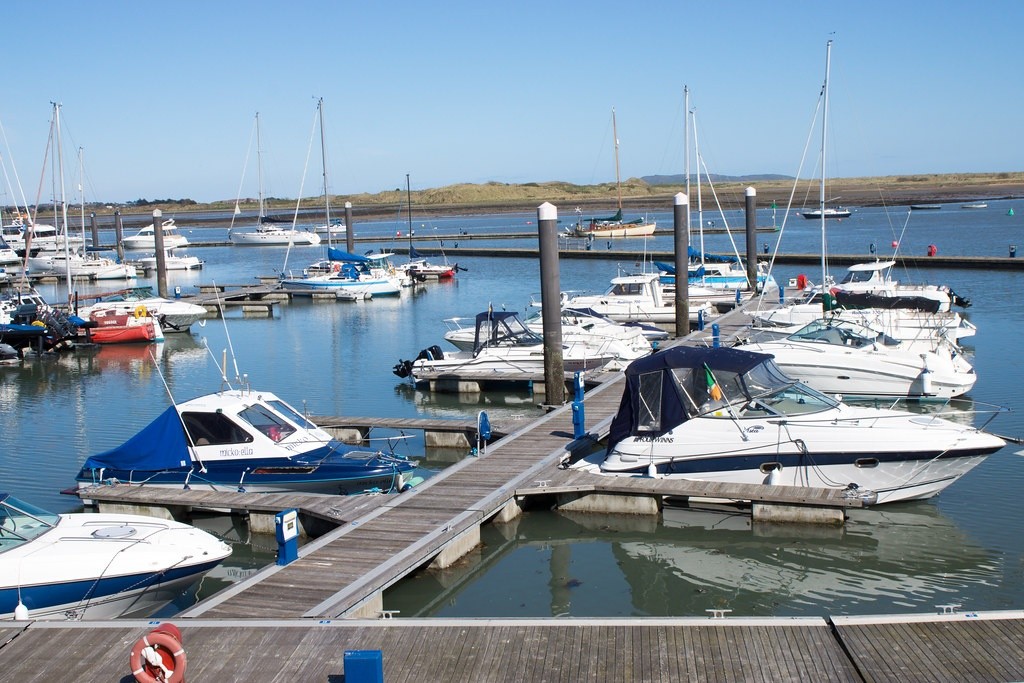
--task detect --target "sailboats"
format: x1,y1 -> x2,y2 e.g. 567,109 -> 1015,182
687,40 -> 976,339
390,172 -> 468,279
615,83 -> 778,290
226,111 -> 323,246
0,101 -> 163,367
802,148 -> 850,219
278,96 -> 402,296
565,105 -> 658,237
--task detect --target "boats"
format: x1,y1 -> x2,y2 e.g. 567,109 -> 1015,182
530,275 -> 714,323
961,204 -> 987,209
565,345 -> 1012,507
139,244 -> 202,271
393,303 -> 653,381
911,205 -> 942,210
356,252 -> 425,286
100,286 -> 207,332
122,217 -> 190,249
803,260 -> 973,312
75,373 -> 418,507
0,492 -> 234,622
314,219 -> 347,233
733,317 -> 977,404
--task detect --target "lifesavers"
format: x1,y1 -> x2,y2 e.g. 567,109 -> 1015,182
129,633 -> 185,683
31,321 -> 46,328
870,243 -> 876,253
607,241 -> 612,249
134,306 -> 146,320
